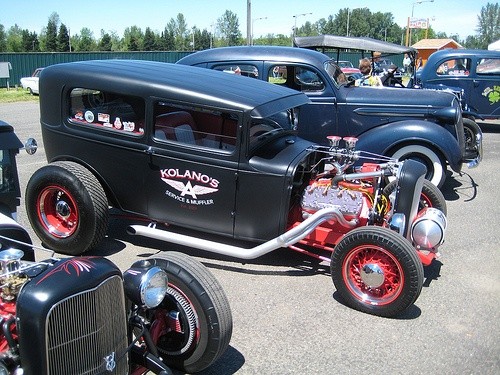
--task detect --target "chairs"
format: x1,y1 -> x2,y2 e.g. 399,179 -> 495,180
136,111 -> 204,146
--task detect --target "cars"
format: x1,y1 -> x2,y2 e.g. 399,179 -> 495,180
222,63 -> 291,81
175,45 -> 469,191
414,44 -> 500,123
20,67 -> 84,96
330,60 -> 360,72
0,118 -> 236,374
25,59 -> 450,318
477,59 -> 499,72
380,59 -> 405,72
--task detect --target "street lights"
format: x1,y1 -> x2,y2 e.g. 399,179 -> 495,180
292,15 -> 296,48
408,0 -> 435,47
346,7 -> 351,36
294,13 -> 313,38
67,25 -> 73,52
384,27 -> 388,42
251,16 -> 267,46
191,25 -> 197,51
210,24 -> 213,49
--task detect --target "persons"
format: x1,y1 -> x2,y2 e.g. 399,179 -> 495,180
273,66 -> 280,79
417,57 -> 423,71
372,52 -> 384,72
234,65 -> 241,75
354,57 -> 382,86
402,54 -> 411,68
